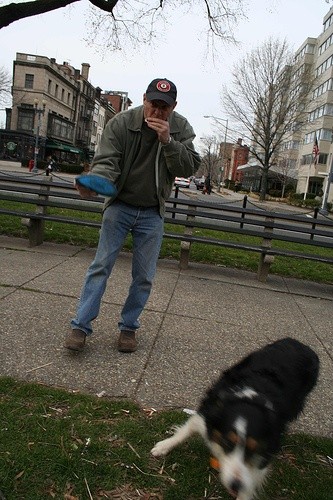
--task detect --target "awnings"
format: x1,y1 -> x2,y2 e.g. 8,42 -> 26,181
48,140 -> 80,153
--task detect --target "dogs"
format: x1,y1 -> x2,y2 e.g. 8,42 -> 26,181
150,337 -> 320,500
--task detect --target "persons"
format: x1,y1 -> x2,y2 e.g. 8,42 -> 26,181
204,177 -> 210,193
48,157 -> 54,173
63,78 -> 202,352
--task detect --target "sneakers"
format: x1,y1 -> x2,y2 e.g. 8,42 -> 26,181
118,330 -> 137,352
66,329 -> 86,352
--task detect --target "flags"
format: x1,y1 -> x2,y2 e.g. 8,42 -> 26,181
313,139 -> 320,155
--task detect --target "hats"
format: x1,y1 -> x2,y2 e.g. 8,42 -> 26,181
146,78 -> 177,106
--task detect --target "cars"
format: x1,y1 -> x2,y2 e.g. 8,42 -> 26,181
174,176 -> 191,188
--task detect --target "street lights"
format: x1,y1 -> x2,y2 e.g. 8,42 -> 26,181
30,96 -> 47,173
202,115 -> 229,194
